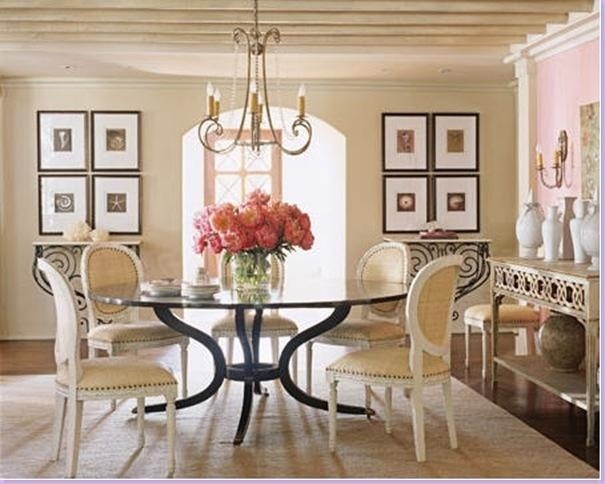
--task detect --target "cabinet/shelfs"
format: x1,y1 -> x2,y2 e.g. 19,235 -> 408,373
485,256 -> 600,447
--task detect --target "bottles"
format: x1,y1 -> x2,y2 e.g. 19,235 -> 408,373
514,196 -> 599,273
192,267 -> 211,286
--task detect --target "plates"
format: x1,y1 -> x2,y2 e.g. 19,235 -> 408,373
137,279 -> 221,300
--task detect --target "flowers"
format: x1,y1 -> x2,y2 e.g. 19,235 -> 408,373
190,188 -> 314,287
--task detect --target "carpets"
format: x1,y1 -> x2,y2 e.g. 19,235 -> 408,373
1,374 -> 600,478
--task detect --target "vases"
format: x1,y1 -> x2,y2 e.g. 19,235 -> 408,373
516,201 -> 546,258
569,200 -> 591,263
557,197 -> 579,259
581,201 -> 600,271
541,205 -> 564,261
230,253 -> 272,299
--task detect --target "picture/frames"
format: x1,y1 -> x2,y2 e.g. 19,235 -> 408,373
382,112 -> 429,172
432,174 -> 480,233
432,112 -> 479,172
90,110 -> 142,172
92,174 -> 142,235
382,174 -> 430,234
36,110 -> 89,172
38,174 -> 90,236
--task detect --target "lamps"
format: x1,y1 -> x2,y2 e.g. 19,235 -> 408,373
197,0 -> 313,155
535,129 -> 568,189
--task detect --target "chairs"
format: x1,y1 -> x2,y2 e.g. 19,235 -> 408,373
212,253 -> 299,388
325,253 -> 458,463
79,244 -> 189,408
304,242 -> 411,405
33,257 -> 178,479
